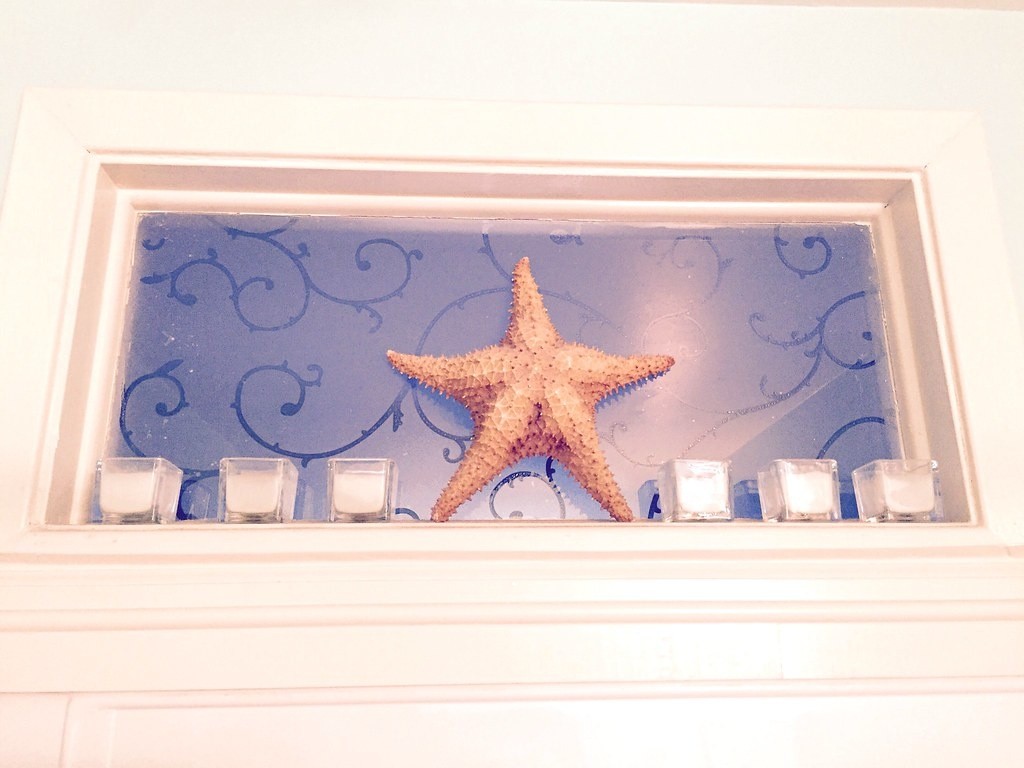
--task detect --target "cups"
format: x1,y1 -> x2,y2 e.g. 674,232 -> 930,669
657,458 -> 735,522
851,458 -> 942,523
327,458 -> 398,524
99,457 -> 183,524
217,458 -> 299,524
756,459 -> 842,521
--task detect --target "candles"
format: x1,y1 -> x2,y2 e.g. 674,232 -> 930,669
99,458 -> 182,523
851,459 -> 942,523
216,457 -> 299,523
326,459 -> 398,523
656,459 -> 731,523
757,459 -> 843,522
